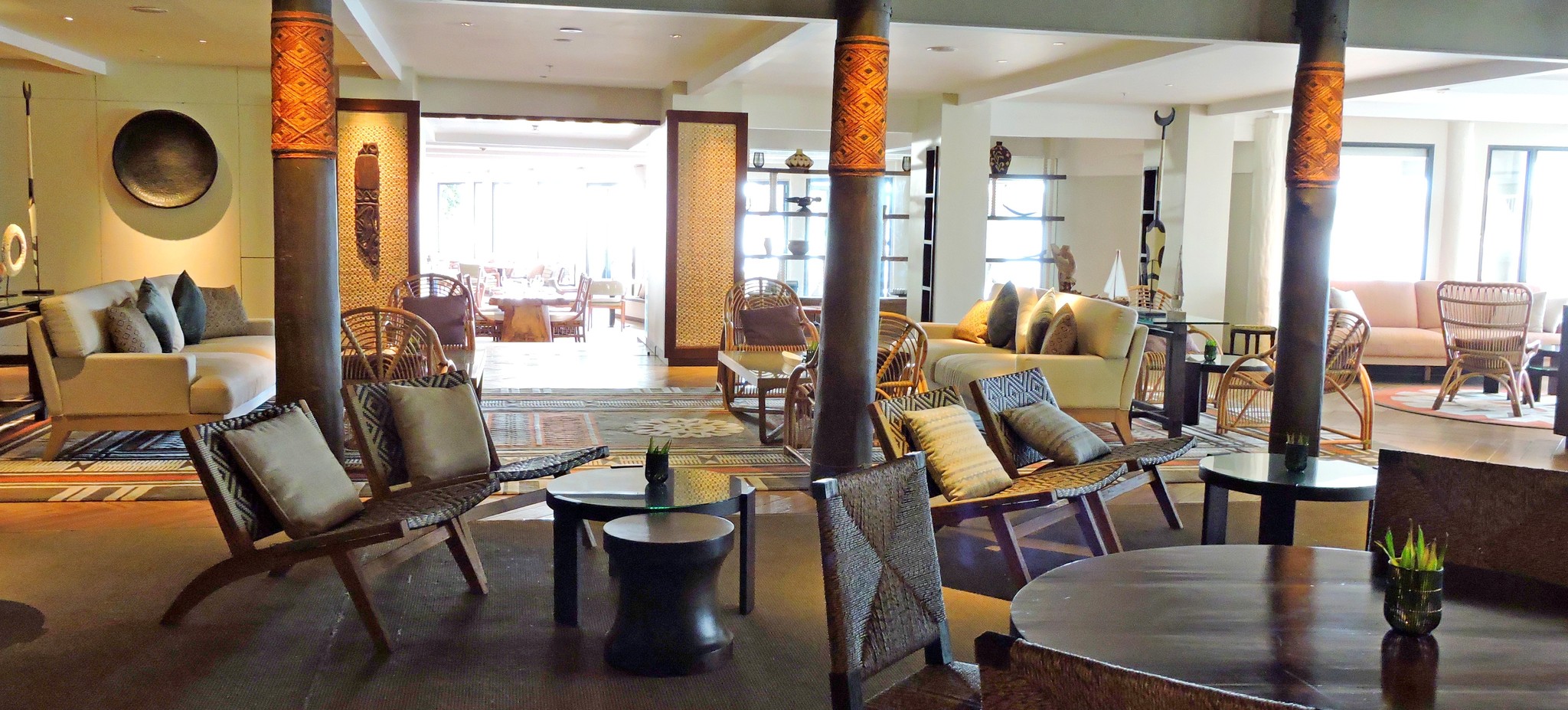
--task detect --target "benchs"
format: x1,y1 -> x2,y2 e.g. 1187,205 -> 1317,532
27,270 -> 276,461
1326,281 -> 1568,383
891,280 -> 1149,445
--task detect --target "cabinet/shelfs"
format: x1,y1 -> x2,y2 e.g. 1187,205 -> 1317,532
744,165 -> 1066,337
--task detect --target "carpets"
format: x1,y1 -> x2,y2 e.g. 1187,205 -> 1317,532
1,385 -> 1405,503
1373,384 -> 1557,429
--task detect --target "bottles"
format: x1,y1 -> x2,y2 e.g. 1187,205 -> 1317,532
764,238 -> 772,255
784,149 -> 814,169
990,142 -> 1012,174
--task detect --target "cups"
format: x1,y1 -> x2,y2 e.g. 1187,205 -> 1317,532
902,156 -> 911,171
883,205 -> 887,214
530,274 -> 545,291
753,152 -> 764,168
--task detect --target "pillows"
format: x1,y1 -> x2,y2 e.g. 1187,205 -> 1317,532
1331,287 -> 1371,331
987,281 -> 1018,347
953,300 -> 999,344
1025,286 -> 1056,354
387,384 -> 491,487
1544,296 -> 1568,333
1486,289 -> 1547,333
1040,301 -> 1077,355
105,270 -> 250,353
1001,399 -> 1110,464
902,405 -> 1013,502
1014,285 -> 1038,353
221,408 -> 366,535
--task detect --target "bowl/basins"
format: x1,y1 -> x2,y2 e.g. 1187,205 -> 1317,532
788,240 -> 809,255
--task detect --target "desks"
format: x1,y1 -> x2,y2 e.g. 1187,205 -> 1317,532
1483,343 -> 1558,402
1199,452 -> 1380,546
1011,540 -> 1567,710
546,463 -> 757,627
1130,307 -> 1229,340
1184,353 -> 1270,424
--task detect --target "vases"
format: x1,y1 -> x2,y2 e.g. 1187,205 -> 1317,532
785,148 -> 814,169
991,141 -> 1011,175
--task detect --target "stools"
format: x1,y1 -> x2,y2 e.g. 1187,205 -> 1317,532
604,509 -> 737,675
1226,325 -> 1276,359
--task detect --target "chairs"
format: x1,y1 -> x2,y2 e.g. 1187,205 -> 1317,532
722,281 -> 1543,709
161,265 -> 625,651
